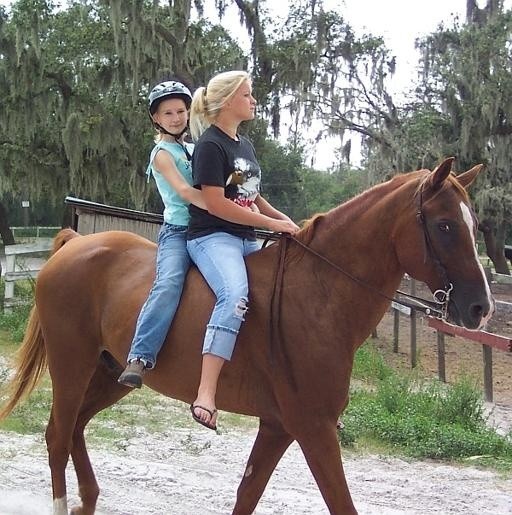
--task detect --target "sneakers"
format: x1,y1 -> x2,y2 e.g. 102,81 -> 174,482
117,359 -> 146,388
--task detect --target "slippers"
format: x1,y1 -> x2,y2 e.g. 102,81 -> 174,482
190,404 -> 217,430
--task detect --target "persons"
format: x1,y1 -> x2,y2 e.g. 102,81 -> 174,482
118,81 -> 260,389
186,72 -> 300,430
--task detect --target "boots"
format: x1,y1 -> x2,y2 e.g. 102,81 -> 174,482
147,81 -> 192,119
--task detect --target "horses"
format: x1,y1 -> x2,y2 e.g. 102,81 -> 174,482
0,155 -> 498,515
